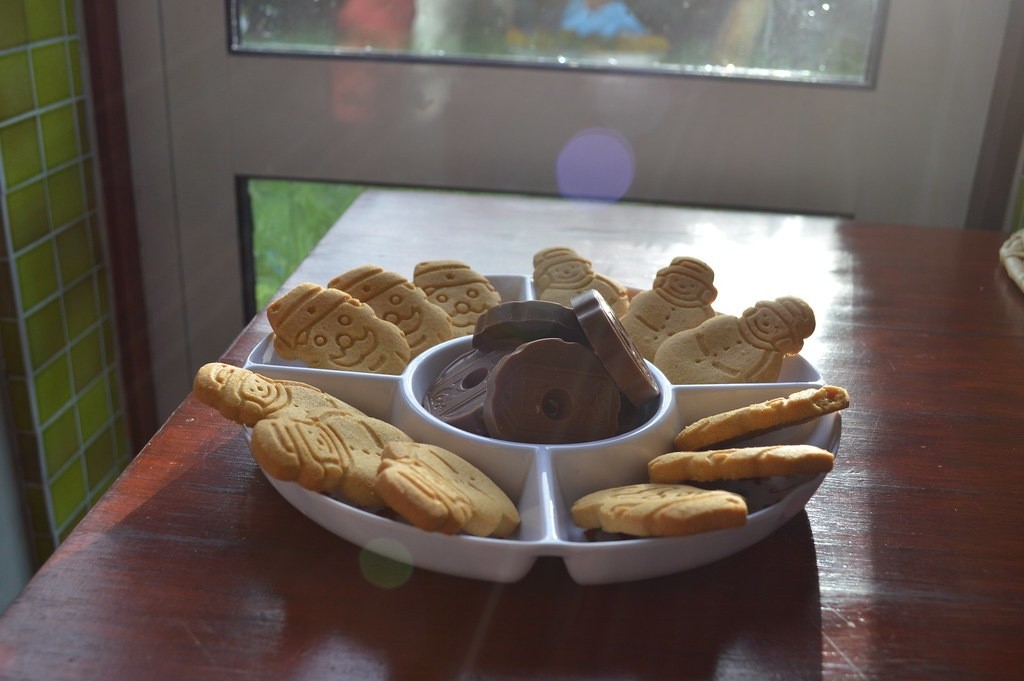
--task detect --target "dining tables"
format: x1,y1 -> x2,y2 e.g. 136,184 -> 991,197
0,187 -> 1024,681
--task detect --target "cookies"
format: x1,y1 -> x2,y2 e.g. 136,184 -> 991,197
194,245 -> 851,538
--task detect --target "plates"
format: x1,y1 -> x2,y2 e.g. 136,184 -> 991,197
242,274 -> 844,586
